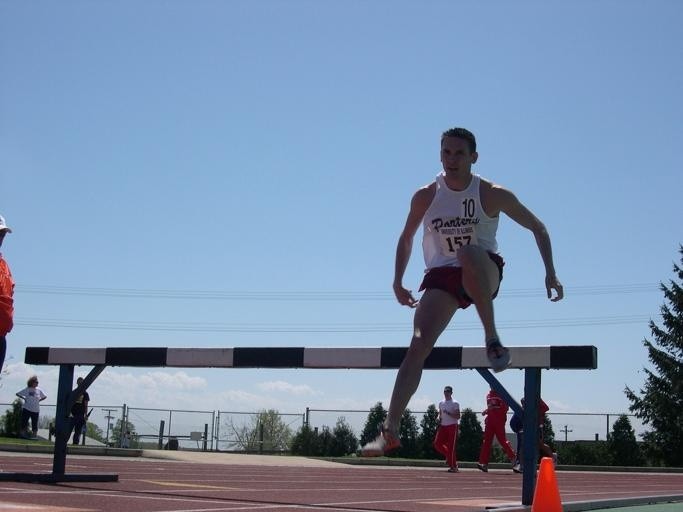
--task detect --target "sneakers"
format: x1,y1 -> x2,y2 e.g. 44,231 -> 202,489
476,463 -> 488,472
552,453 -> 557,466
361,432 -> 401,457
448,468 -> 459,472
513,464 -> 523,473
486,342 -> 512,373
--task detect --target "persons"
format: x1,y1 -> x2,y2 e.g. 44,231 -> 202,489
66,377 -> 89,445
16,377 -> 47,440
0,213 -> 14,372
121,431 -> 131,448
509,397 -> 525,466
433,386 -> 460,473
513,398 -> 558,473
362,128 -> 564,458
476,384 -> 517,472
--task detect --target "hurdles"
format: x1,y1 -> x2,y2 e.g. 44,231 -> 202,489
0,346 -> 598,510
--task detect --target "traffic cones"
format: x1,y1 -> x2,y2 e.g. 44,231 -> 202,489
530,457 -> 563,512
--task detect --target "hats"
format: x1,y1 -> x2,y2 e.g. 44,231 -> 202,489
0,217 -> 11,232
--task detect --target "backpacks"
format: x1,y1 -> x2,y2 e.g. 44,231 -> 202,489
510,412 -> 521,433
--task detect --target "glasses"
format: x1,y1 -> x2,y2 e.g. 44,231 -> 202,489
444,390 -> 452,393
35,381 -> 38,384
